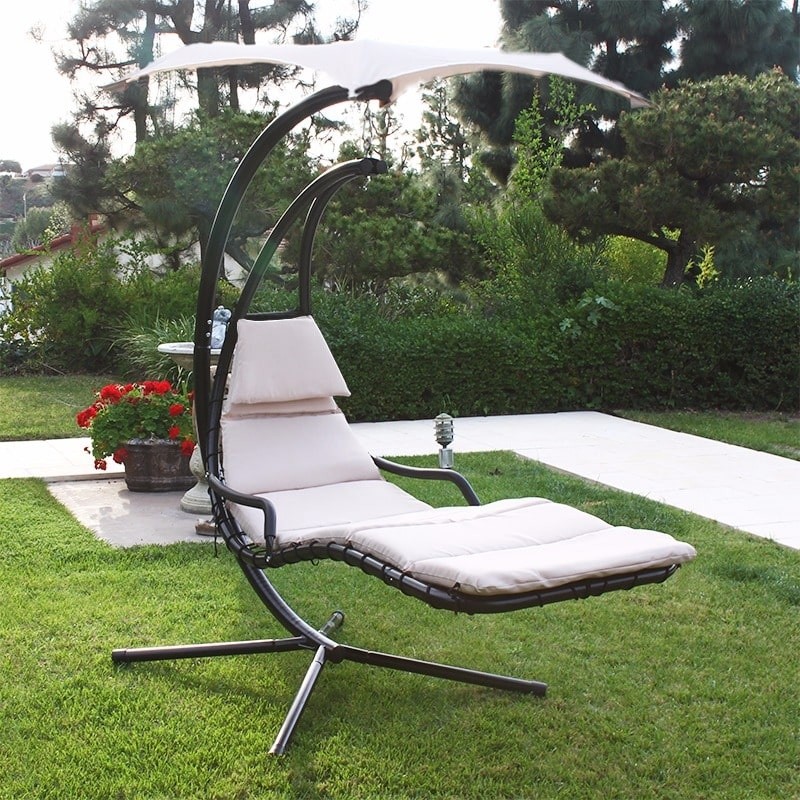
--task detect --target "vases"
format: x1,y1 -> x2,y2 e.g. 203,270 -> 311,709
117,438 -> 197,492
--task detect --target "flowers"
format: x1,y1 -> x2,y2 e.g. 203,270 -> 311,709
76,378 -> 194,471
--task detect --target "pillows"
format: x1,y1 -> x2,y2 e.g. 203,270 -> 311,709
227,316 -> 352,405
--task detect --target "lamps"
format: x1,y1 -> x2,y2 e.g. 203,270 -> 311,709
434,409 -> 456,466
